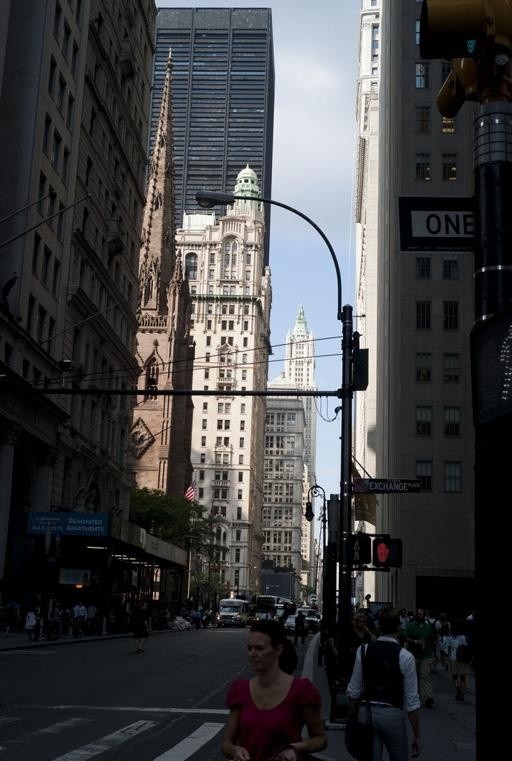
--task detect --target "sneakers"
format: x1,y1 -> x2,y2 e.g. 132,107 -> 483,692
454,691 -> 466,701
420,698 -> 435,708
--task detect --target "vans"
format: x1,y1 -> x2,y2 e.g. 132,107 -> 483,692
216,599 -> 248,628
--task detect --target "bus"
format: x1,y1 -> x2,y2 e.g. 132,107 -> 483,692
256,595 -> 296,632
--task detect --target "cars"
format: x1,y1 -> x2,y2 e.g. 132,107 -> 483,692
284,608 -> 321,633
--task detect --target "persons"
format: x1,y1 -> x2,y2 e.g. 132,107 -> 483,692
22,600 -> 218,654
294,606 -> 474,760
219,619 -> 328,760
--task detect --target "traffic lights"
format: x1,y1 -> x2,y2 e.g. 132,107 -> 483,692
342,530 -> 402,572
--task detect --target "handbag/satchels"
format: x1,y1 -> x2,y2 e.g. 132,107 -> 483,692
343,715 -> 376,760
455,643 -> 471,663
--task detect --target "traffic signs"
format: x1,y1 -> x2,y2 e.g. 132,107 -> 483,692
353,477 -> 423,493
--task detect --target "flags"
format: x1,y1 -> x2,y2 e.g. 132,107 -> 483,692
184,478 -> 198,503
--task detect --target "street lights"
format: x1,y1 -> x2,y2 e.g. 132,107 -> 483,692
195,189 -> 355,684
305,485 -> 327,564
